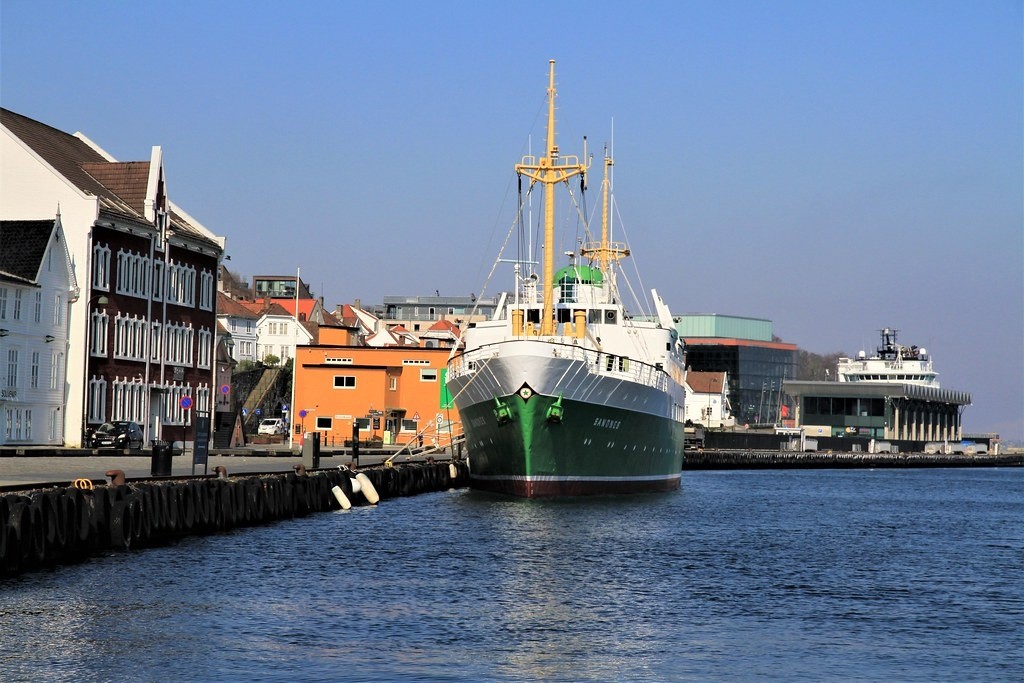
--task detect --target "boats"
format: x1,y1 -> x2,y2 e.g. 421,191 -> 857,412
441,60 -> 688,504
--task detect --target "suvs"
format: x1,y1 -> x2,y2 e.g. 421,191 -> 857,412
91,420 -> 142,456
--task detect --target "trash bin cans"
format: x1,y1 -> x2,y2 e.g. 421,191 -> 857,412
150,439 -> 172,478
302,431 -> 321,468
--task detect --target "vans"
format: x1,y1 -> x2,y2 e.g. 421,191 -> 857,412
257,418 -> 283,436
